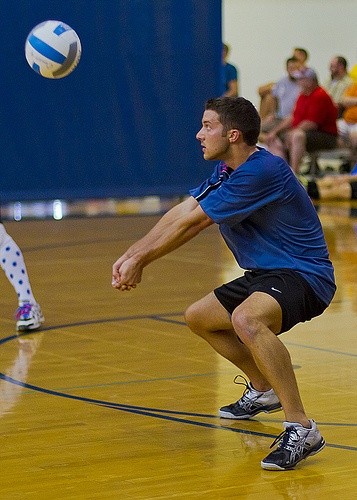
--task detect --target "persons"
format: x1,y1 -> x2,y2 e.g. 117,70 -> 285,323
222,43 -> 238,97
113,97 -> 338,471
0,222 -> 44,331
258,48 -> 356,212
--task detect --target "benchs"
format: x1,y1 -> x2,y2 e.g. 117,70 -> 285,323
308,149 -> 351,173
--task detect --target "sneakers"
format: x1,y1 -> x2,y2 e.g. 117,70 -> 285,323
262,417 -> 326,471
219,374 -> 284,421
17,301 -> 44,331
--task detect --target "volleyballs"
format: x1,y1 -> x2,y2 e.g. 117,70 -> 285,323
25,20 -> 82,79
